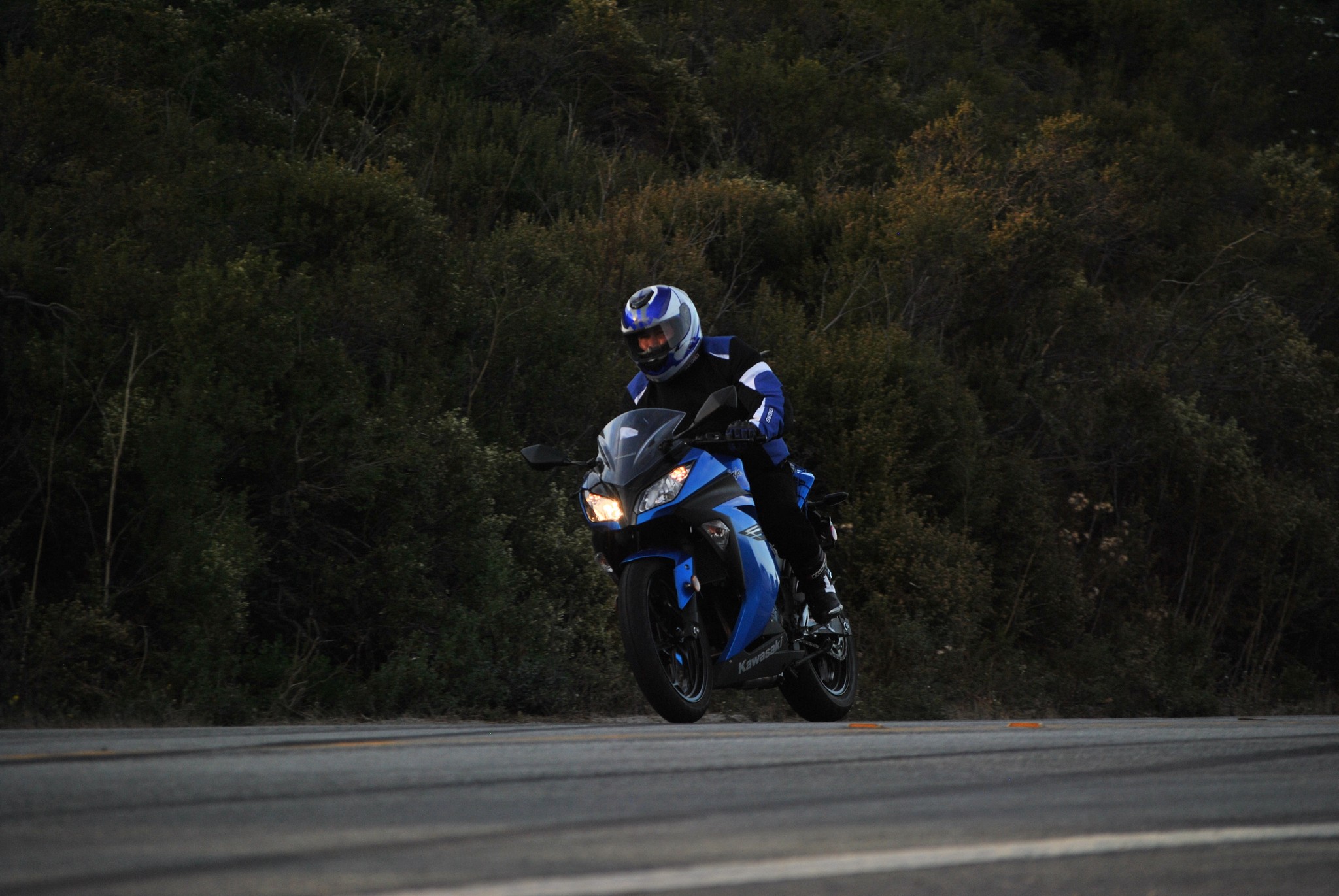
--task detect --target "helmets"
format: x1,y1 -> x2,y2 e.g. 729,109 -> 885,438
621,284 -> 702,382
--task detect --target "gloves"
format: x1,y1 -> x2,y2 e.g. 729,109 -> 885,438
725,420 -> 761,447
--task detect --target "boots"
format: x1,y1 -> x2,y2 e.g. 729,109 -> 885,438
793,542 -> 843,624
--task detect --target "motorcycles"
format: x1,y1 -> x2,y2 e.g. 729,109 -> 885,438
517,385 -> 861,725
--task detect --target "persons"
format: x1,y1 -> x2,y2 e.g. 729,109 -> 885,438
604,284 -> 840,630
